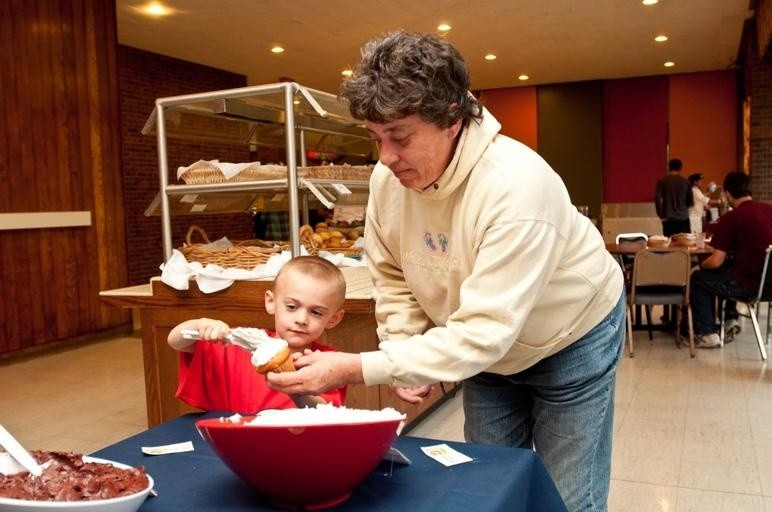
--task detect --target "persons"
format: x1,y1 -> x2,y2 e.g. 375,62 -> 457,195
688,163 -> 768,352
655,156 -> 696,235
266,34 -> 629,512
687,168 -> 723,232
166,253 -> 345,411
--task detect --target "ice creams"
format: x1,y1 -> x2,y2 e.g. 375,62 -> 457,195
252,339 -> 296,376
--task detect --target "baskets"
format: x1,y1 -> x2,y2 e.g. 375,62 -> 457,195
300,237 -> 360,257
180,224 -> 281,273
178,163 -> 288,184
298,163 -> 381,182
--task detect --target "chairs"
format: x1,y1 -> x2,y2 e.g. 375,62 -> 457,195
718,246 -> 772,361
615,233 -> 648,282
628,250 -> 697,358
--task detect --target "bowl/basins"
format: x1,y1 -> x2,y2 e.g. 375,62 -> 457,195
194,413 -> 408,509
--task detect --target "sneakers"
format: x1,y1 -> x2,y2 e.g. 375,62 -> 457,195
684,333 -> 721,348
724,320 -> 740,344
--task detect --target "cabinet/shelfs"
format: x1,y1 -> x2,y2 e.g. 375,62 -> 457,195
143,84 -> 376,270
98,274 -> 460,438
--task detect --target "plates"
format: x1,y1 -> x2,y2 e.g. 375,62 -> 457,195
0,455 -> 155,512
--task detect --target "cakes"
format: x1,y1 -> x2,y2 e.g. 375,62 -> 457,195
313,222 -> 367,250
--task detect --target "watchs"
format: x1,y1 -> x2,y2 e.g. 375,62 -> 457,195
698,261 -> 702,269
